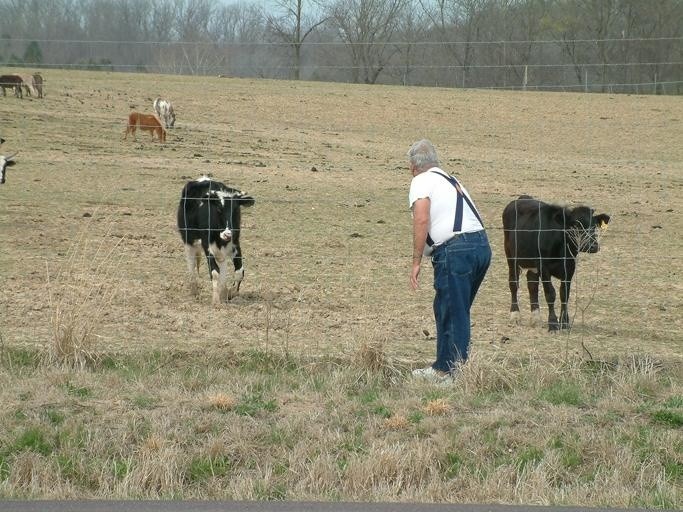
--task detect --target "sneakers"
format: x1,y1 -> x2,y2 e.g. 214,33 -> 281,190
411,365 -> 453,387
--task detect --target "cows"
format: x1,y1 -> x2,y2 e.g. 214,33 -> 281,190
123,111 -> 166,144
0,151 -> 19,184
177,174 -> 255,311
153,96 -> 177,130
0,72 -> 43,101
501,193 -> 610,337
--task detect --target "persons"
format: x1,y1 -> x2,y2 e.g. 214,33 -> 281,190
405,138 -> 493,388
